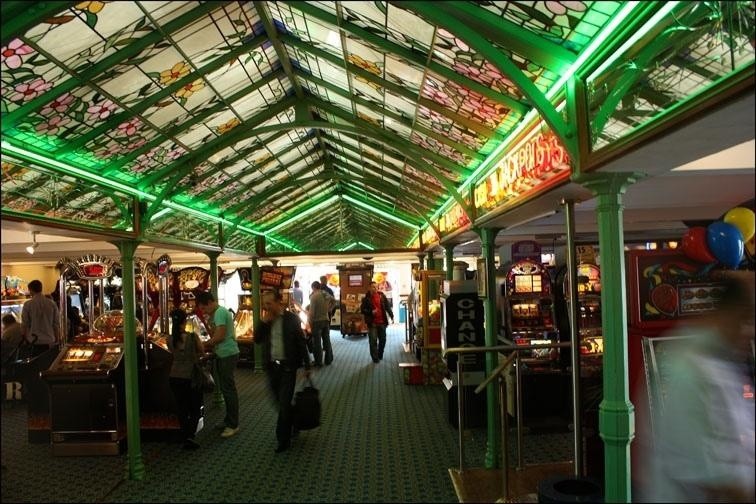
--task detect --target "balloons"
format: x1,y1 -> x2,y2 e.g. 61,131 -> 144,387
374,273 -> 383,284
706,223 -> 744,269
329,275 -> 339,285
681,226 -> 716,263
723,208 -> 755,244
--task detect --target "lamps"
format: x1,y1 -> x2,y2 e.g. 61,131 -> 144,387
25,231 -> 39,254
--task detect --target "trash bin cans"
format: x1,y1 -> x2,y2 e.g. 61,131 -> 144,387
399,299 -> 408,322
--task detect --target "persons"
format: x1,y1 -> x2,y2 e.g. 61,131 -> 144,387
255,292 -> 313,452
137,294 -> 161,332
360,281 -> 394,363
293,276 -> 336,366
43,286 -> 122,339
0,280 -> 60,367
167,293 -> 240,451
639,268 -> 755,504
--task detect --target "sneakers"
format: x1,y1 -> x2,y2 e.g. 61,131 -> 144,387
275,443 -> 291,454
215,421 -> 239,438
372,353 -> 384,363
183,439 -> 200,450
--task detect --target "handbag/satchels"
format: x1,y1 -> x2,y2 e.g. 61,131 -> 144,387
292,387 -> 321,431
191,363 -> 215,394
